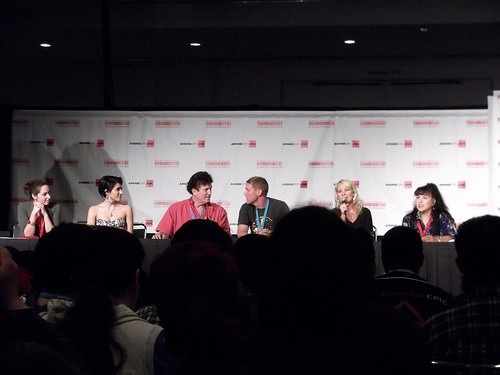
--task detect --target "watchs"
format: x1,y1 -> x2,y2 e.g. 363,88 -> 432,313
27,221 -> 36,226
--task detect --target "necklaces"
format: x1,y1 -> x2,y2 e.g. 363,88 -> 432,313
103,203 -> 116,217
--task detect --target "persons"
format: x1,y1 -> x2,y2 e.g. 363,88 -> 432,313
18,177 -> 55,237
0,206 -> 500,375
402,183 -> 457,242
152,171 -> 230,239
331,179 -> 373,239
87,175 -> 133,234
237,176 -> 290,238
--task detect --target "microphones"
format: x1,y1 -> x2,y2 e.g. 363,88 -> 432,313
342,198 -> 347,215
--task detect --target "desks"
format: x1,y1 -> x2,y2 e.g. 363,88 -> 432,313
0,237 -> 38,250
373,242 -> 462,293
137,239 -> 170,265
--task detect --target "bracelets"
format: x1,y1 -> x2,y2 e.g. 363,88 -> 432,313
438,236 -> 441,242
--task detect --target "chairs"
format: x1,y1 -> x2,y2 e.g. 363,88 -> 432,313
133,223 -> 146,239
